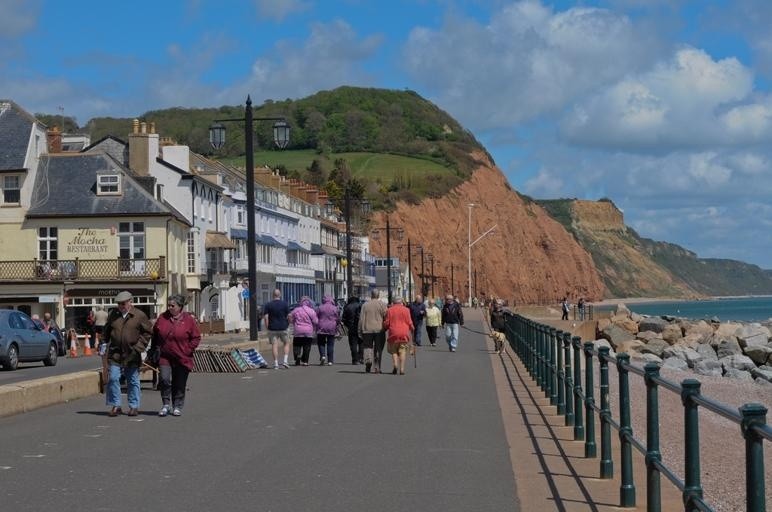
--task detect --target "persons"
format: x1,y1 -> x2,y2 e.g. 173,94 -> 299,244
100,291 -> 153,416
151,294 -> 202,417
258,289 -> 465,375
472,297 -> 478,310
90,304 -> 108,350
561,297 -> 570,320
578,297 -> 585,319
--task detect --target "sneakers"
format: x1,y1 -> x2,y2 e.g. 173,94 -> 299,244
450,347 -> 455,352
295,361 -> 310,366
282,362 -> 290,369
352,358 -> 364,365
158,405 -> 171,417
320,356 -> 326,366
392,367 -> 406,375
416,342 -> 422,346
430,343 -> 437,346
365,360 -> 372,373
172,407 -> 182,416
328,361 -> 333,366
273,365 -> 279,369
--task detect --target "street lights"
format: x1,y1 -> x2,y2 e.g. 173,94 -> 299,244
467,203 -> 498,307
209,93 -> 292,342
397,237 -> 482,307
371,214 -> 404,310
324,187 -> 376,304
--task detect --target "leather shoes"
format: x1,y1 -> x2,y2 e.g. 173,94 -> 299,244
127,408 -> 138,416
109,406 -> 122,417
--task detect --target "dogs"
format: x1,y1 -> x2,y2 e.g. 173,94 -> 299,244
489,330 -> 506,355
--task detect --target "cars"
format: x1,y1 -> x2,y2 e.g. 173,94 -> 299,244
1,309 -> 59,370
26,319 -> 68,357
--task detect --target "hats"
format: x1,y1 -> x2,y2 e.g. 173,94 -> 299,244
114,291 -> 132,302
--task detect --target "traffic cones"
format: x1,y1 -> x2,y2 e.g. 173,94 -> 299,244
66,330 -> 81,358
93,332 -> 100,354
80,332 -> 95,357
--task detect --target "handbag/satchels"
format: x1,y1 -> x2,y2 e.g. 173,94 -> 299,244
334,321 -> 349,341
101,355 -> 109,385
565,304 -> 570,312
145,344 -> 161,369
